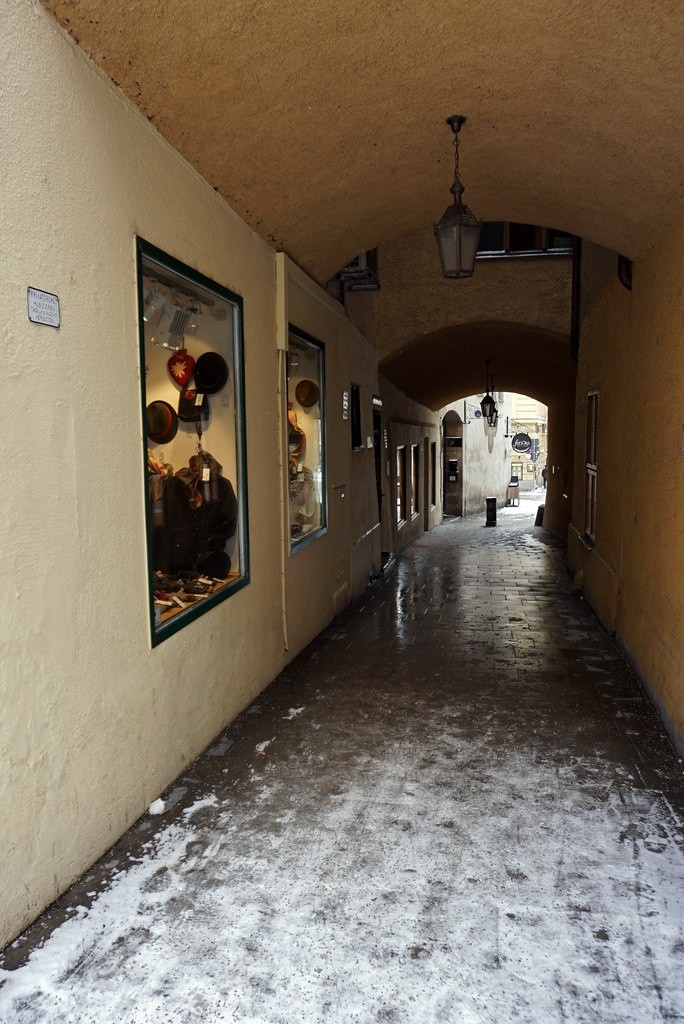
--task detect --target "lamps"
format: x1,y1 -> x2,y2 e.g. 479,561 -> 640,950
487,386 -> 498,427
433,113 -> 481,279
480,361 -> 495,417
